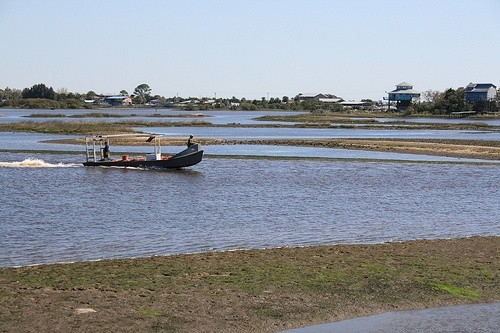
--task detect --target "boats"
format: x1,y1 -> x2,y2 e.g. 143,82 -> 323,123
81,133 -> 204,170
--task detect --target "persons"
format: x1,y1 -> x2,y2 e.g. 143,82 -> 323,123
188,134 -> 195,148
103,141 -> 110,159
